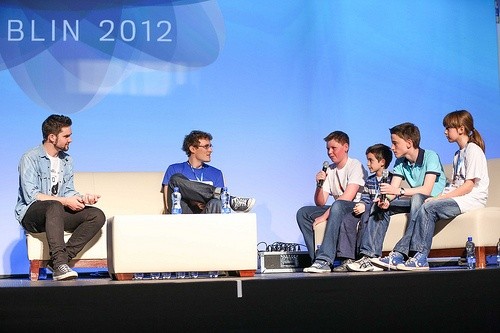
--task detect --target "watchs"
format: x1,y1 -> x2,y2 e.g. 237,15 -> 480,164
399,187 -> 405,196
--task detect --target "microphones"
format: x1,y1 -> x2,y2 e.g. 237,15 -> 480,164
318,161 -> 329,187
381,170 -> 389,202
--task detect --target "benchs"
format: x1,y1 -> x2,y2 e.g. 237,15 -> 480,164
25,171 -> 165,282
313,159 -> 500,269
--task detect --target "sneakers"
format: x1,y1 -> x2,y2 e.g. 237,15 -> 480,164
303,259 -> 331,273
230,196 -> 255,213
333,260 -> 357,272
47,261 -> 78,281
396,253 -> 430,272
346,255 -> 384,272
371,251 -> 405,271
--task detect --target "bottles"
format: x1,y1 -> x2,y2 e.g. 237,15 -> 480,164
134,270 -> 218,280
466,237 -> 476,270
171,187 -> 182,214
497,239 -> 500,268
221,187 -> 231,213
316,245 -> 321,255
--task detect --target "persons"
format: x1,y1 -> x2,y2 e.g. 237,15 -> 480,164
14,113 -> 106,281
370,107 -> 490,271
295,130 -> 368,274
346,121 -> 446,272
332,143 -> 394,272
160,130 -> 257,213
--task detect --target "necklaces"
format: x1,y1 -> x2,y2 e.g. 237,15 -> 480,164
196,163 -> 203,169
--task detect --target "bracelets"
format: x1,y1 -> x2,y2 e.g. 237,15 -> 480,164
386,197 -> 392,204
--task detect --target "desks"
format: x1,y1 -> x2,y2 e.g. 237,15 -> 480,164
106,213 -> 258,282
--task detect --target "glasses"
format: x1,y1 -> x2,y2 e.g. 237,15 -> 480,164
195,143 -> 213,149
